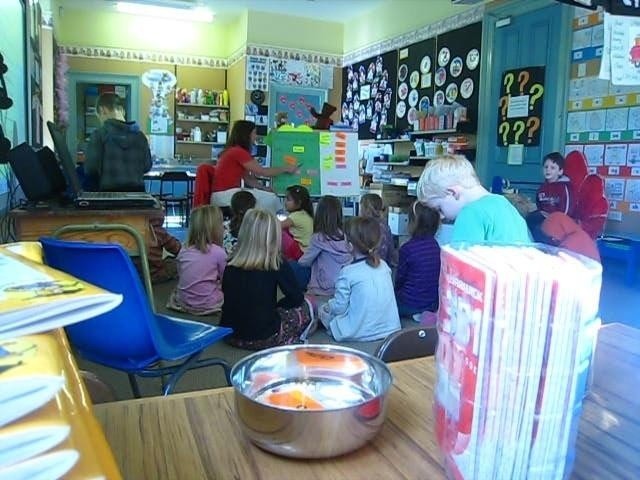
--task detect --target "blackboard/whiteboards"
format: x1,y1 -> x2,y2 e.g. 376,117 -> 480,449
272,131 -> 360,198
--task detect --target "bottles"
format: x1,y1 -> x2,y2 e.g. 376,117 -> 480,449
189,87 -> 229,107
193,127 -> 203,142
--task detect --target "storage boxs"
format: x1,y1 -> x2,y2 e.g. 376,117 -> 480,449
388,211 -> 409,236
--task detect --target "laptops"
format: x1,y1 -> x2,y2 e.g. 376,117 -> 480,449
47,121 -> 161,209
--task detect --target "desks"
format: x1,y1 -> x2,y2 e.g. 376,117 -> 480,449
144,171 -> 197,209
90,323 -> 640,480
8,198 -> 176,395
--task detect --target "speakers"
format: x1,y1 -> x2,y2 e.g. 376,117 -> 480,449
35,146 -> 67,197
7,142 -> 52,202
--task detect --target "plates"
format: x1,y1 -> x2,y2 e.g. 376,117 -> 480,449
251,89 -> 265,105
445,82 -> 457,103
395,56 -> 431,124
435,68 -> 447,86
437,47 -> 451,67
449,57 -> 463,77
460,79 -> 474,98
432,89 -> 445,107
466,49 -> 481,70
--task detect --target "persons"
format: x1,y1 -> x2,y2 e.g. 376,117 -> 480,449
280,185 -> 315,260
175,205 -> 228,313
360,194 -> 393,266
84,93 -> 153,192
417,154 -> 533,251
393,201 -> 441,318
133,210 -> 181,284
318,216 -> 402,342
278,195 -> 354,310
503,150 -> 608,263
210,121 -> 297,211
222,190 -> 257,257
218,208 -> 318,352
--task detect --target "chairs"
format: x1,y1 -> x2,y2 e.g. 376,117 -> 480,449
375,326 -> 437,362
160,171 -> 189,227
38,237 -> 234,397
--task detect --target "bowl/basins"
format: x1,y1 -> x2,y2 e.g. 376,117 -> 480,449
228,343 -> 393,462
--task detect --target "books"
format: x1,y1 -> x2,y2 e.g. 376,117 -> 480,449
432,241 -> 604,480
1,233 -> 126,480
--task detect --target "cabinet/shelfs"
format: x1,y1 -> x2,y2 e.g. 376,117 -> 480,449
176,101 -> 228,146
370,120 -> 475,188
